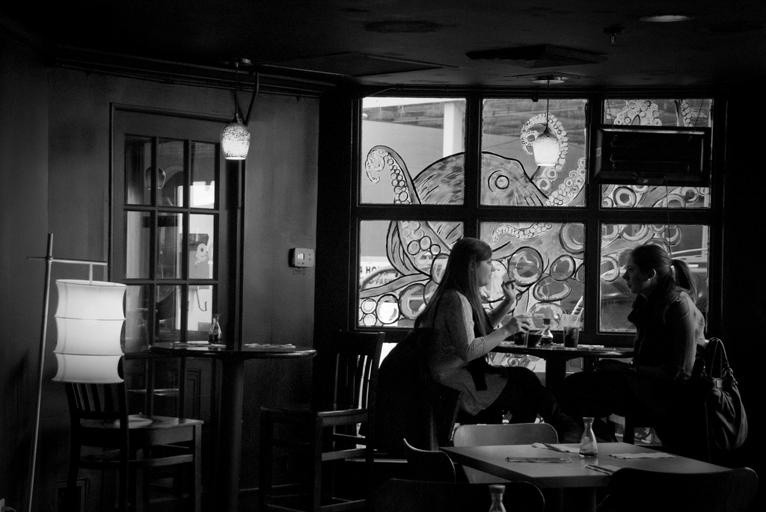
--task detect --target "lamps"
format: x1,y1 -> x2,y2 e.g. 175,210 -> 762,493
219,54 -> 251,162
29,232 -> 126,511
145,165 -> 166,192
528,76 -> 566,168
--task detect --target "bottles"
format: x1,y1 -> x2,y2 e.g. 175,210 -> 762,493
539,317 -> 554,346
579,418 -> 598,456
208,315 -> 223,347
487,486 -> 506,511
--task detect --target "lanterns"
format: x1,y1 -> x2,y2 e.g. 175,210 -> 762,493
49,278 -> 126,385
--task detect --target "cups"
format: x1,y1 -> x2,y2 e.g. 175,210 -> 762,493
513,326 -> 529,347
562,315 -> 580,348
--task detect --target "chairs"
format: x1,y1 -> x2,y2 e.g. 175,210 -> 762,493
623,337 -> 732,458
256,328 -> 384,511
402,422 -> 560,510
602,466 -> 765,509
391,330 -> 464,446
49,354 -> 202,512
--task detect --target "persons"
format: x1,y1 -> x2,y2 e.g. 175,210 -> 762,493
415,238 -> 568,442
559,245 -> 706,418
